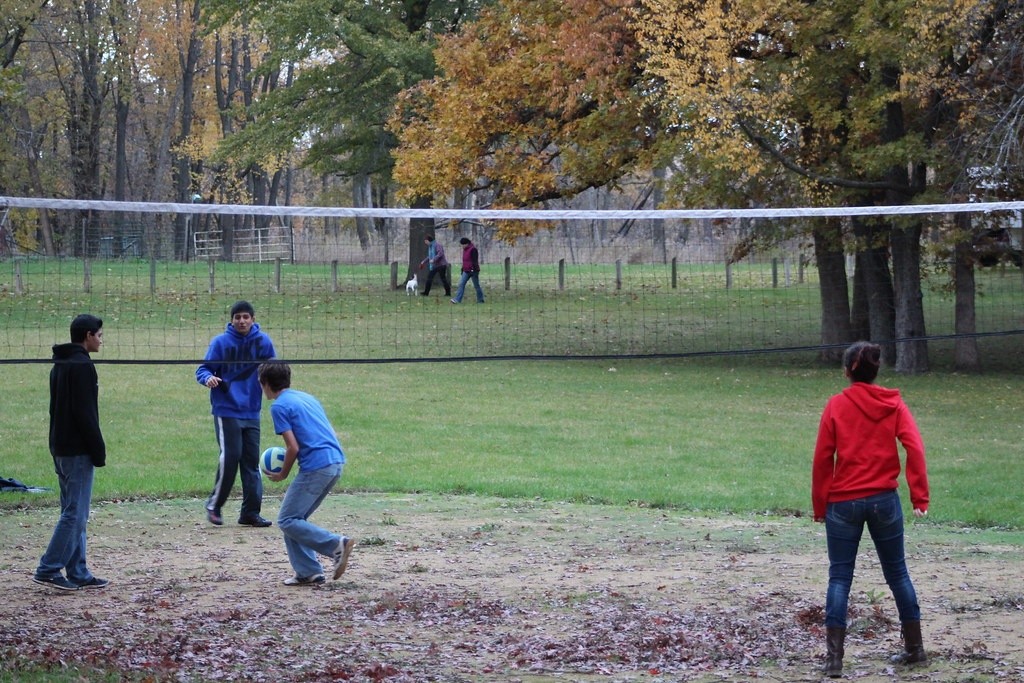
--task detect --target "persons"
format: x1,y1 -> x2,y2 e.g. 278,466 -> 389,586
258,357 -> 355,585
450,237 -> 484,303
810,341 -> 929,677
419,234 -> 452,296
32,314 -> 108,593
196,300 -> 276,528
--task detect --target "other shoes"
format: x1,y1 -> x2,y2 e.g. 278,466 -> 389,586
450,299 -> 457,304
444,294 -> 451,297
419,292 -> 429,296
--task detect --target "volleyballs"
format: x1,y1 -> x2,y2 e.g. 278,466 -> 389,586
260,447 -> 288,476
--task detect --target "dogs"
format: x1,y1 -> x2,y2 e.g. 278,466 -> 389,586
405,273 -> 418,296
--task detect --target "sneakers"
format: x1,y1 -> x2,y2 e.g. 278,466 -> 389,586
330,537 -> 354,580
32,576 -> 79,591
67,576 -> 109,590
206,506 -> 223,526
281,572 -> 326,586
238,513 -> 272,527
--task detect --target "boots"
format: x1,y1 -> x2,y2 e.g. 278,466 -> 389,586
821,626 -> 845,676
890,621 -> 927,665
